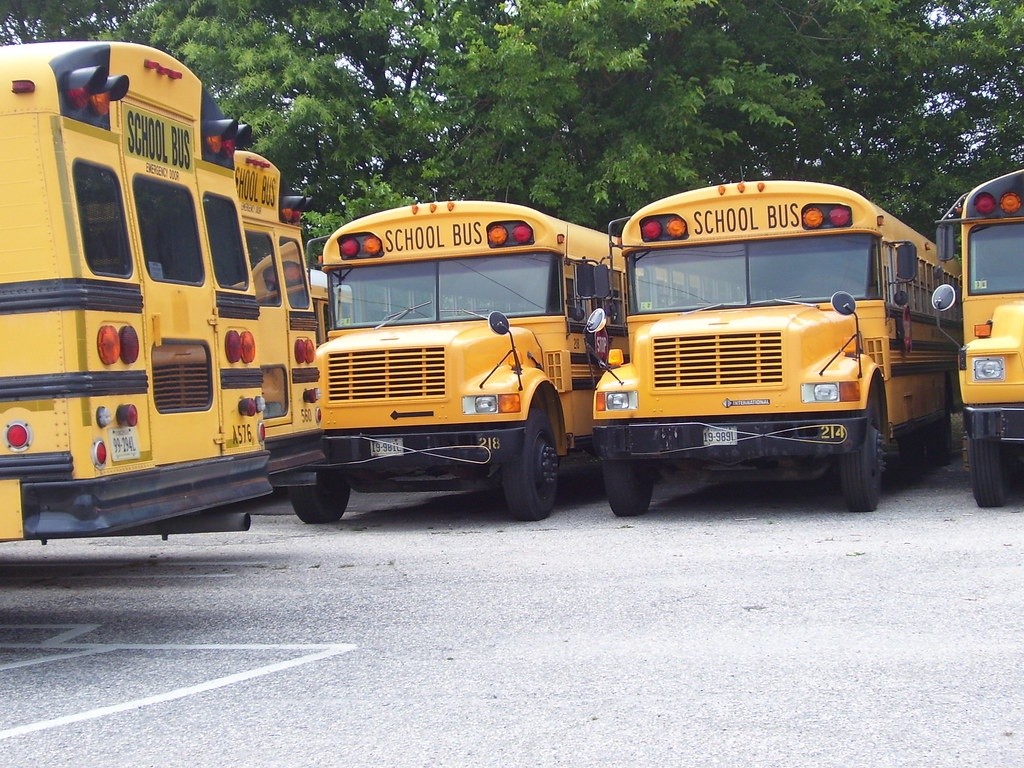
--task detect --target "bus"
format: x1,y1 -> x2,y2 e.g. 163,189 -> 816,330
2,37 -> 352,544
285,193 -> 626,525
586,180 -> 964,514
939,171 -> 1024,512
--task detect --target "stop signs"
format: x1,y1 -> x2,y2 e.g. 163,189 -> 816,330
593,326 -> 611,367
903,303 -> 912,353
